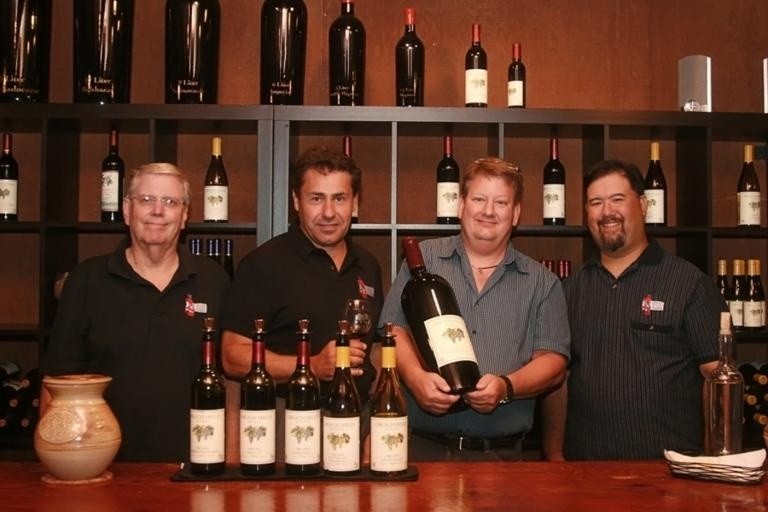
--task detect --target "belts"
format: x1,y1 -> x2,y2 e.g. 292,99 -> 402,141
412,427 -> 525,451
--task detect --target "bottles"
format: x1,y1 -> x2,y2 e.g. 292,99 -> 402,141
737,144 -> 761,228
0,132 -> 19,224
285,318 -> 322,475
738,360 -> 768,449
329,1 -> 366,106
259,2 -> 308,105
713,256 -> 767,342
465,24 -> 488,108
537,257 -> 572,281
507,43 -> 526,108
370,322 -> 407,476
163,1 -> 222,103
342,135 -> 359,224
401,237 -> 482,413
204,137 -> 229,223
436,134 -> 461,224
71,1 -> 135,103
542,138 -> 566,225
1,1 -> 53,105
186,237 -> 235,286
0,359 -> 42,437
100,130 -> 125,223
322,318 -> 363,476
395,7 -> 425,106
701,311 -> 744,455
642,142 -> 667,227
189,317 -> 225,475
239,318 -> 276,476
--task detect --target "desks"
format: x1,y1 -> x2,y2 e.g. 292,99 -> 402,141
2,449 -> 767,512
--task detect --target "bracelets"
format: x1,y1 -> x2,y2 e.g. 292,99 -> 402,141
498,374 -> 514,406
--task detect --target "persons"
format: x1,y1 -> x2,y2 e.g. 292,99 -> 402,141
370,156 -> 573,462
219,144 -> 385,468
37,160 -> 230,463
540,158 -> 736,462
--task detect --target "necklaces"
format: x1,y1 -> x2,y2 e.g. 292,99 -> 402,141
470,263 -> 498,272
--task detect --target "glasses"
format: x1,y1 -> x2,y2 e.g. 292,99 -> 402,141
131,194 -> 186,208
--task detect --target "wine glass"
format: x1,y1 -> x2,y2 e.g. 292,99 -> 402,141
344,298 -> 371,342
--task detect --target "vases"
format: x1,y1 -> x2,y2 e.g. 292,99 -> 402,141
30,372 -> 122,486
